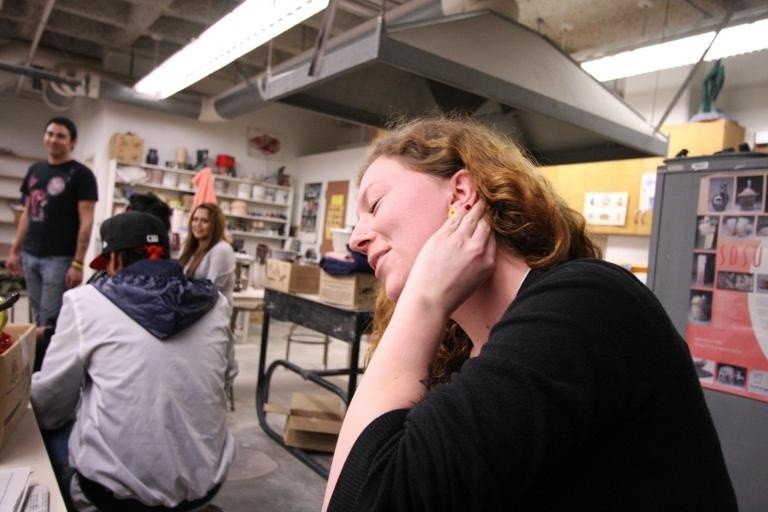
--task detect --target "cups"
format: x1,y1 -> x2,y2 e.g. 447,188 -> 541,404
256,244 -> 271,263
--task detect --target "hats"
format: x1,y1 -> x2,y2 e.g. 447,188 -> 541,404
89,210 -> 170,270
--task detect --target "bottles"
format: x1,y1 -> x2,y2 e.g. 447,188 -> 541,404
241,265 -> 249,288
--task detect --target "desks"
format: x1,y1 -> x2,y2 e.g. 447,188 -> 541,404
1,397 -> 68,512
256,289 -> 373,482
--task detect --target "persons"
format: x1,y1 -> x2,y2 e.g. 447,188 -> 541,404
8,117 -> 99,374
90,193 -> 236,309
321,115 -> 739,512
29,210 -> 239,512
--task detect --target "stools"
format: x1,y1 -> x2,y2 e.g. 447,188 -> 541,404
196,504 -> 225,512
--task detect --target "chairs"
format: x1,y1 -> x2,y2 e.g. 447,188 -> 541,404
283,320 -> 330,371
224,307 -> 240,412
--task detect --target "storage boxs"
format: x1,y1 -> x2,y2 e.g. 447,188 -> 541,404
319,266 -> 382,308
109,132 -> 144,165
262,393 -> 348,454
265,260 -> 318,294
0,323 -> 36,456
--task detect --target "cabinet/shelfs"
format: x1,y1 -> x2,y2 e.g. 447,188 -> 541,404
106,160 -> 294,290
0,151 -> 47,292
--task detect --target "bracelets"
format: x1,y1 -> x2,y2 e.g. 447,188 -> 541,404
71,260 -> 83,270
